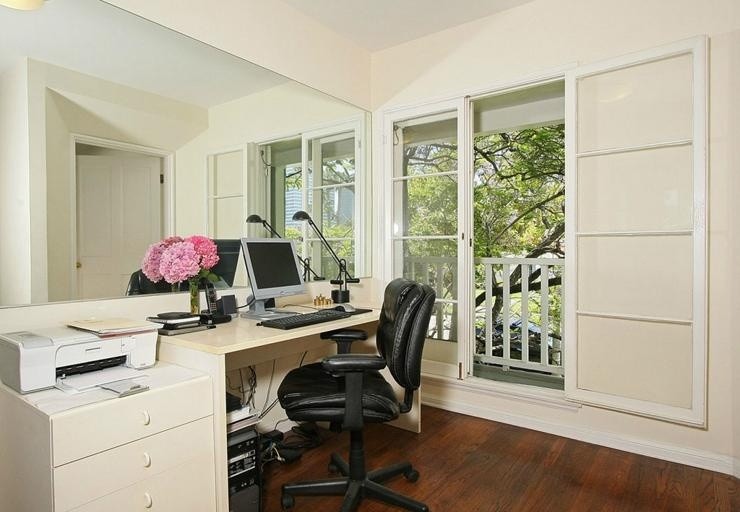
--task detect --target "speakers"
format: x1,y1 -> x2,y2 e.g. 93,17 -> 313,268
222,295 -> 237,318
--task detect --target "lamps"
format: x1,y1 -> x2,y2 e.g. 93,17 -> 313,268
293,212 -> 359,303
246,215 -> 325,281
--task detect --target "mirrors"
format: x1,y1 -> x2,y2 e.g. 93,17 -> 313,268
0,0 -> 371,309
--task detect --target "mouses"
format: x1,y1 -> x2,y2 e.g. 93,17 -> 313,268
335,304 -> 356,312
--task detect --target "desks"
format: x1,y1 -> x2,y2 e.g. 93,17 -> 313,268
156,303 -> 421,512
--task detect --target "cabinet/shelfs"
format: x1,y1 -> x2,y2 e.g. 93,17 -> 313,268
0,360 -> 214,512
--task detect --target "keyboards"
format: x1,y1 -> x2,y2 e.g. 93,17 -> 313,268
258,310 -> 351,329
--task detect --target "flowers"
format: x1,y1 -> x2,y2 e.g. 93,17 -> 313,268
141,236 -> 183,291
160,236 -> 220,313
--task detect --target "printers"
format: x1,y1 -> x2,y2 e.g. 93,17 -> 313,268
0,317 -> 158,397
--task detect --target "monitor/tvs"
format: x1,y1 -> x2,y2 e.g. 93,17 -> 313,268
241,237 -> 307,322
182,239 -> 241,290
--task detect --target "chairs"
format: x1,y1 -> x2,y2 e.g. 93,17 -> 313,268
125,269 -> 168,294
278,278 -> 436,511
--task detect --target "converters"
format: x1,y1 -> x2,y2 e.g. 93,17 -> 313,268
258,430 -> 283,449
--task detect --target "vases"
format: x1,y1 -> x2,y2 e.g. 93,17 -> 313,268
188,279 -> 200,314
172,283 -> 179,292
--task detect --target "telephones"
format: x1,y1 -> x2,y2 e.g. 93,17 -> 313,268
202,283 -> 231,325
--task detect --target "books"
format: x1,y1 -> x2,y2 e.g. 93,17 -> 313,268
147,312 -> 207,335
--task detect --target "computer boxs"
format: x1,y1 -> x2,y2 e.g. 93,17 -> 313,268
227,428 -> 262,512
